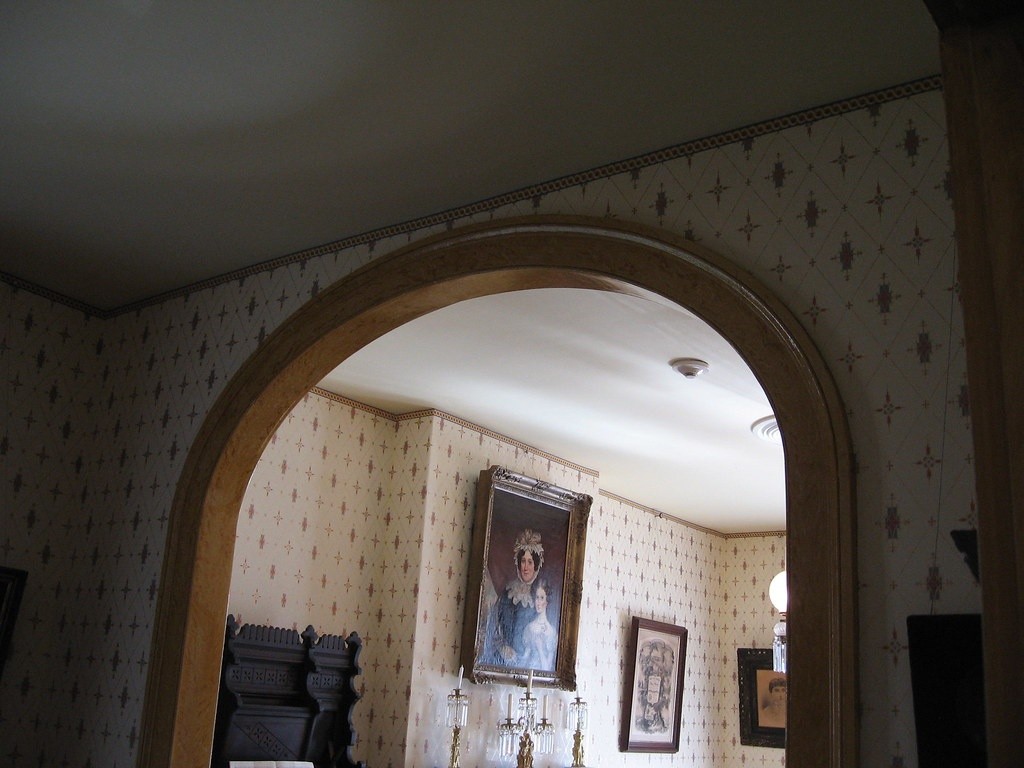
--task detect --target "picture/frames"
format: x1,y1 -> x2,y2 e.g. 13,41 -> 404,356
617,615 -> 688,753
736,647 -> 785,748
461,465 -> 595,688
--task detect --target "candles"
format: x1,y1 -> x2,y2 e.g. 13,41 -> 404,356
457,664 -> 464,689
544,694 -> 548,718
527,669 -> 534,692
507,694 -> 512,718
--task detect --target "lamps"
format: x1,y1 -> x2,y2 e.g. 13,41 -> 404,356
769,569 -> 787,645
